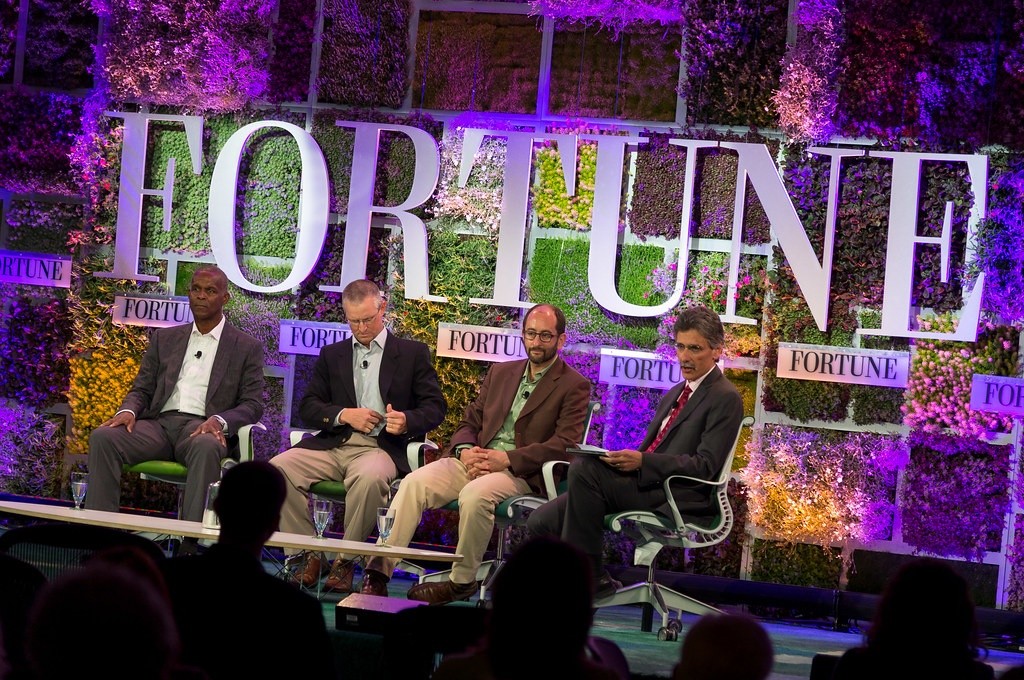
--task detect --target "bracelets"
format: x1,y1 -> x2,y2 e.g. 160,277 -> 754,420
214,416 -> 225,430
456,448 -> 470,460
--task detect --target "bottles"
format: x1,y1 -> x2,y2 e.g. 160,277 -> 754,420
202,480 -> 222,530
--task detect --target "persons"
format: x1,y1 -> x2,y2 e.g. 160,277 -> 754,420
267,280 -> 448,594
0,460 -> 339,680
84,265 -> 264,556
839,557 -> 996,680
526,306 -> 744,600
434,536 -> 630,680
680,610 -> 773,680
360,299 -> 591,606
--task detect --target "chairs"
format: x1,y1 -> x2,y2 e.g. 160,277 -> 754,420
123,418 -> 757,642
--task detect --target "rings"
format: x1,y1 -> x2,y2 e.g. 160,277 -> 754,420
616,463 -> 620,467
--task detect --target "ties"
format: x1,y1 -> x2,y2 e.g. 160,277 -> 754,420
645,385 -> 693,453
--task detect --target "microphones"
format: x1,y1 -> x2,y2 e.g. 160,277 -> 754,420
363,361 -> 368,369
525,391 -> 529,398
671,401 -> 679,407
197,351 -> 202,357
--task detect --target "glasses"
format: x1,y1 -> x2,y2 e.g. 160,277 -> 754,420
522,330 -> 559,342
345,305 -> 380,324
673,342 -> 712,353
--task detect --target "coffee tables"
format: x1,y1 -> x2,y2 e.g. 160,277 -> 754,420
1,500 -> 465,599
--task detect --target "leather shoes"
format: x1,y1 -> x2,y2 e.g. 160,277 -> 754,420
290,550 -> 328,588
360,575 -> 389,598
325,557 -> 354,592
408,579 -> 478,602
592,570 -> 616,599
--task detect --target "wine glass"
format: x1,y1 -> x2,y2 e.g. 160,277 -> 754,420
374,507 -> 396,549
70,471 -> 88,511
311,499 -> 333,539
